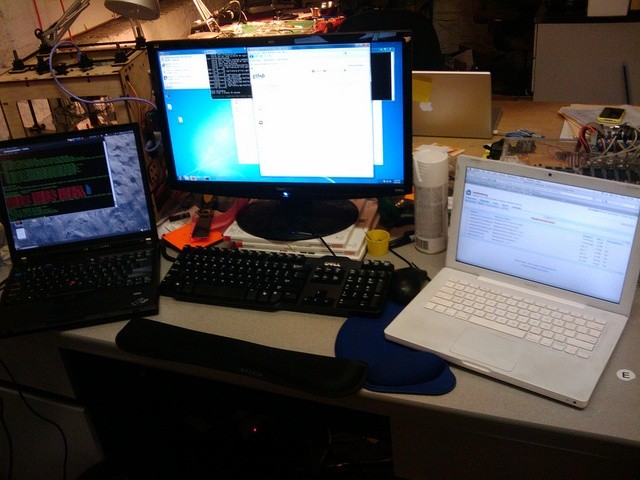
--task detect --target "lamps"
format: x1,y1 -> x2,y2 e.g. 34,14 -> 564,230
34,0 -> 160,52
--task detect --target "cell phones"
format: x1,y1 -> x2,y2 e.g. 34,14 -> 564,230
597,106 -> 627,125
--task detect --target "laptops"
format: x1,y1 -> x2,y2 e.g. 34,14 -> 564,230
384,155 -> 640,409
0,120 -> 162,337
409,69 -> 502,139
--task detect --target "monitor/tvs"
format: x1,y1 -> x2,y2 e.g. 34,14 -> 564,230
148,27 -> 414,242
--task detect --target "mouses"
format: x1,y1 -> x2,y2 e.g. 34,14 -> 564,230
391,266 -> 426,306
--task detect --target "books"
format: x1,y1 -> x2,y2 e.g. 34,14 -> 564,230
223,197 -> 382,263
559,102 -> 640,142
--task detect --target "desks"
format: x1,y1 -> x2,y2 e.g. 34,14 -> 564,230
188,12 -> 364,39
1,92 -> 639,480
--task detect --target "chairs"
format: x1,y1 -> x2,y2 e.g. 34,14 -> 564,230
491,19 -> 528,72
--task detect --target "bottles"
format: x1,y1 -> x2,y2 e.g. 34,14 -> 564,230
412,149 -> 450,256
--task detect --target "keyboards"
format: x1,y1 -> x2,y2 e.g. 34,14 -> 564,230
160,244 -> 397,319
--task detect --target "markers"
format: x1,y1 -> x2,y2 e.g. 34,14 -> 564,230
388,234 -> 415,248
